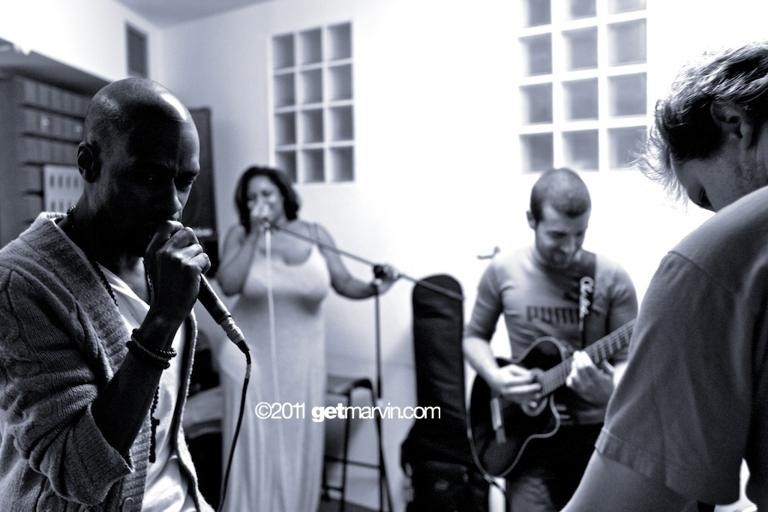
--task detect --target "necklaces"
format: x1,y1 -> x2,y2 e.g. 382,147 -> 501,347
65,207 -> 176,464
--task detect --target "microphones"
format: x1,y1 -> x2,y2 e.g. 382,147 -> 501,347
169,228 -> 249,352
262,217 -> 272,234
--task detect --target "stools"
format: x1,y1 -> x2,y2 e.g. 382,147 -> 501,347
320,371 -> 386,512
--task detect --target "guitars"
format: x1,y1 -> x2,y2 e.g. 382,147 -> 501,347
469,318 -> 637,476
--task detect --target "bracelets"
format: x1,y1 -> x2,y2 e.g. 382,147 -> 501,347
125,323 -> 177,369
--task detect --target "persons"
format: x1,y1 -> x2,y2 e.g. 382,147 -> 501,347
216,167 -> 401,512
0,76 -> 223,512
463,168 -> 642,512
558,43 -> 768,512
